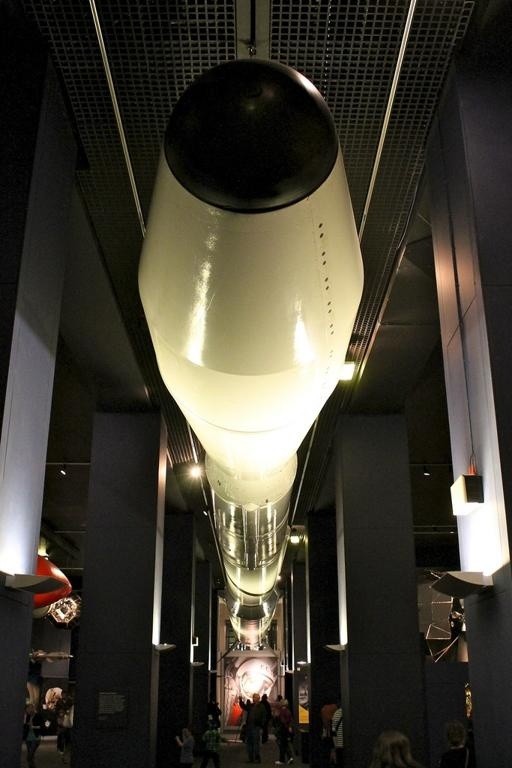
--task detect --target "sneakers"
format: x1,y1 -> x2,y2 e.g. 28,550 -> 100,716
274,760 -> 285,765
287,757 -> 294,765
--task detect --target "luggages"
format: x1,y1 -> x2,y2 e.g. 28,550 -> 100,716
239,724 -> 247,743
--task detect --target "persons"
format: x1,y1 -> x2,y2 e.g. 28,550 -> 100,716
238,695 -> 251,743
57,688 -> 74,756
321,701 -> 338,753
200,720 -> 223,768
202,692 -> 222,735
258,693 -> 272,745
326,707 -> 344,768
275,695 -> 283,707
23,704 -> 41,768
175,725 -> 196,768
237,692 -> 267,764
436,721 -> 478,768
368,729 -> 423,768
272,699 -> 295,766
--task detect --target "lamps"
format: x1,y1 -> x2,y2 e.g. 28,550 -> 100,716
191,662 -> 204,667
429,569 -> 495,599
326,645 -> 346,652
154,643 -> 177,653
5,573 -> 68,596
